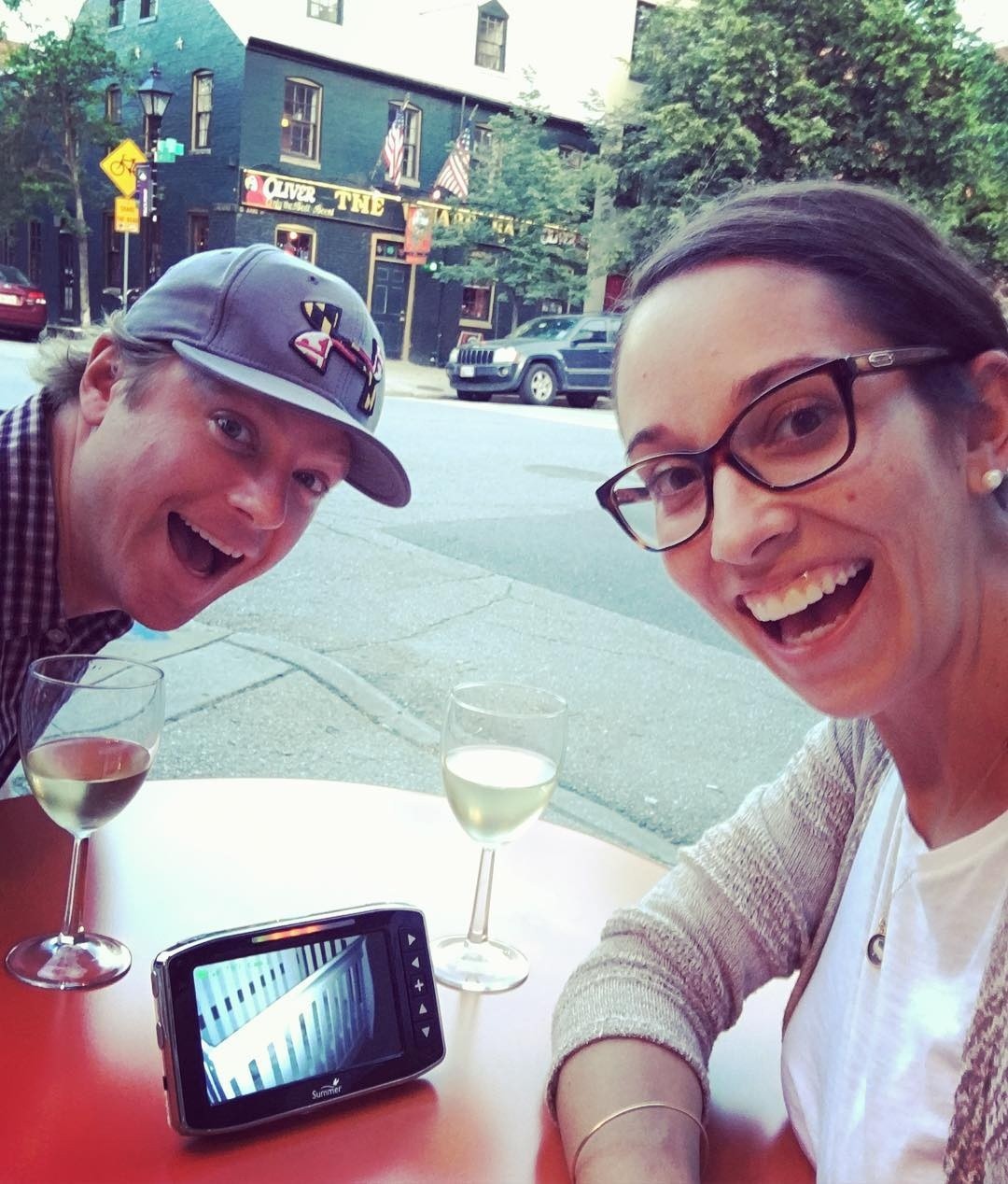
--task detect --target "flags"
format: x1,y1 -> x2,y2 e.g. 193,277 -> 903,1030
435,125 -> 471,203
383,108 -> 403,190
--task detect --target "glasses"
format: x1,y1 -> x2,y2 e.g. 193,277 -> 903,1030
594,345 -> 963,552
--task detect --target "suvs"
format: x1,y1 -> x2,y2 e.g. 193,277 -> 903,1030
444,314 -> 623,408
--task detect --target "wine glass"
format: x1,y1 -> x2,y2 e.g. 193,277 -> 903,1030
424,683 -> 567,993
3,654 -> 167,991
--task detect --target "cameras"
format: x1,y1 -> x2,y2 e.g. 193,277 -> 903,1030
152,903 -> 445,1135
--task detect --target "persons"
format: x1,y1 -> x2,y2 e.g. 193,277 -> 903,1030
0,242 -> 412,798
546,182 -> 1008,1184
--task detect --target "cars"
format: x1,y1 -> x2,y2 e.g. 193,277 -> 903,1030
0,264 -> 47,341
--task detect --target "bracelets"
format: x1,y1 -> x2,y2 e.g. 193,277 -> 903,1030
572,1103 -> 711,1184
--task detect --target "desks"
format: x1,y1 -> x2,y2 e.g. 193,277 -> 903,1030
0,774 -> 817,1184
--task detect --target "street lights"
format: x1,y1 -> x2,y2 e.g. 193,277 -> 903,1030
134,62 -> 173,288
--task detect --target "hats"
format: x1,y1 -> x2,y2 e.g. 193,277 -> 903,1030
122,243 -> 411,508
